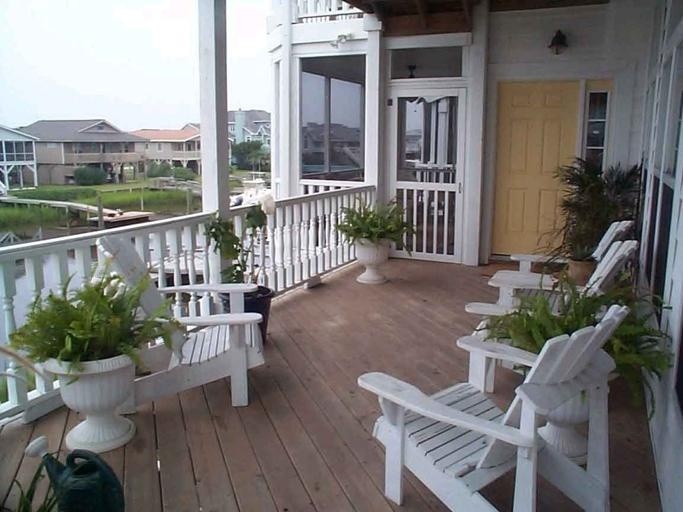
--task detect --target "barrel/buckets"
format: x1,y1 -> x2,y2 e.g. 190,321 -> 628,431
217,285 -> 274,346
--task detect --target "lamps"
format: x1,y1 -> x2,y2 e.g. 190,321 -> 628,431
547,29 -> 568,54
401,65 -> 419,103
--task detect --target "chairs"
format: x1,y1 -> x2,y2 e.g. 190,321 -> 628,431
79,239 -> 265,408
457,239 -> 641,393
358,306 -> 632,512
483,221 -> 634,316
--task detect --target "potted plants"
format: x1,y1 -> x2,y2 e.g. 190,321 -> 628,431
202,211 -> 274,346
474,254 -> 674,464
8,251 -> 187,457
532,155 -> 642,285
333,198 -> 415,285
